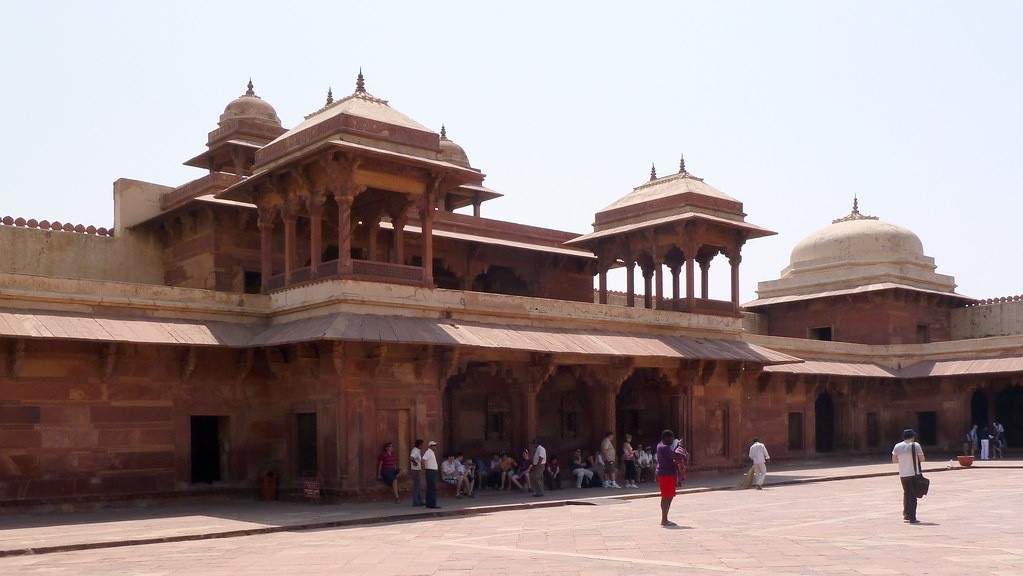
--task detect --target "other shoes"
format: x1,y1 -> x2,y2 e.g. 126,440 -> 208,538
904,516 -> 909,520
755,485 -> 763,490
395,498 -> 400,501
631,484 -> 639,488
430,506 -> 442,509
455,494 -> 464,499
625,484 -> 632,488
468,495 -> 478,498
576,486 -> 582,489
532,494 -> 543,497
604,484 -> 612,488
910,520 -> 920,523
611,483 -> 622,488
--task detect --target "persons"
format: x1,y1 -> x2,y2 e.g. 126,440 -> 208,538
531,438 -> 547,497
570,448 -> 594,489
601,431 -> 622,489
410,439 -> 425,507
669,436 -> 683,450
623,434 -> 639,488
377,443 -> 406,504
892,429 -> 925,524
422,440 -> 443,509
546,455 -> 561,491
441,451 -> 486,500
748,437 -> 770,491
656,429 -> 680,527
636,443 -> 659,483
990,420 -> 1007,458
586,449 -> 607,488
488,448 -> 533,491
963,424 -> 978,455
980,427 -> 994,460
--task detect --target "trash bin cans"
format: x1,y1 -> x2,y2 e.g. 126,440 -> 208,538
258,474 -> 276,501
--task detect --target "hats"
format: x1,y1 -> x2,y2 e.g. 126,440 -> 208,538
902,429 -> 917,436
531,440 -> 538,444
428,441 -> 438,447
645,446 -> 652,451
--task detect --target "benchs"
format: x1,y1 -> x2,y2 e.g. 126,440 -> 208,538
476,461 -> 504,489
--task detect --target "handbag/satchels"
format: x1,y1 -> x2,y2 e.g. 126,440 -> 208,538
912,476 -> 930,498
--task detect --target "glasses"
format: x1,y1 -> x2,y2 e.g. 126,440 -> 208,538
576,451 -> 581,452
637,447 -> 643,448
387,446 -> 394,447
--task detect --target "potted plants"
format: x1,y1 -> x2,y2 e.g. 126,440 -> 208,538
956,443 -> 974,466
